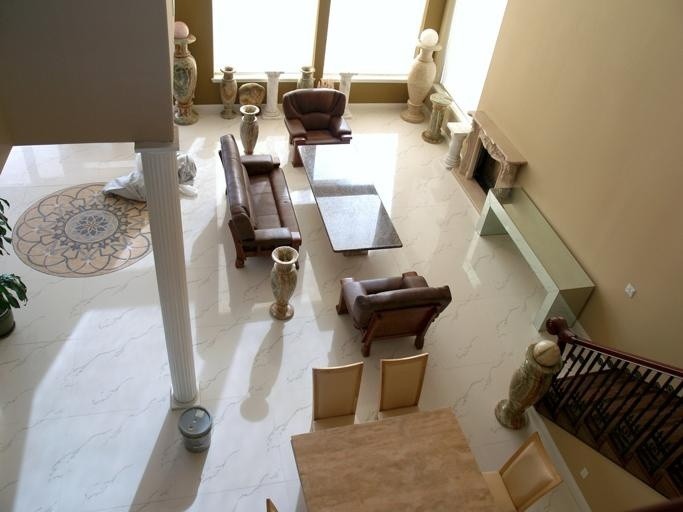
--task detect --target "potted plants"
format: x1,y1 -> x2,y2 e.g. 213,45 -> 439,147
0,197 -> 30,340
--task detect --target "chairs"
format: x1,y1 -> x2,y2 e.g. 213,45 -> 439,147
479,430 -> 563,512
309,351 -> 429,431
336,271 -> 452,358
281,87 -> 353,167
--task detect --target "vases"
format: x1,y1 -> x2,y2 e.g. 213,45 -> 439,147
220,65 -> 259,154
268,245 -> 299,322
297,65 -> 317,89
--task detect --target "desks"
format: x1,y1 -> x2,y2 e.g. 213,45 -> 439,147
291,406 -> 496,512
476,186 -> 596,334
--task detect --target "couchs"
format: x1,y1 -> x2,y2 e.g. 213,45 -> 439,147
218,133 -> 302,271
